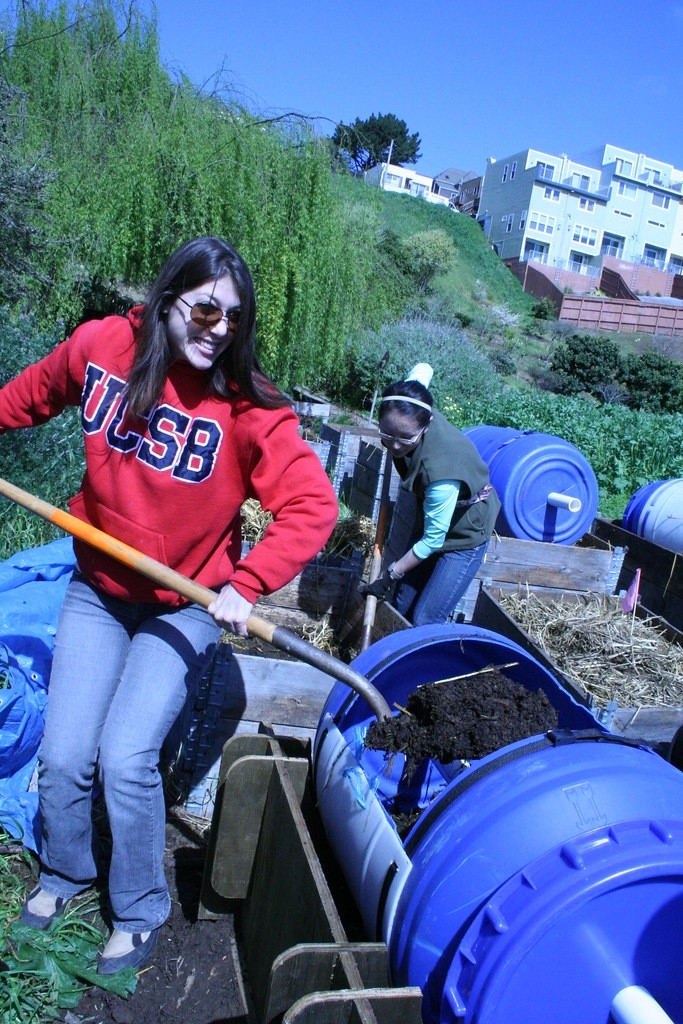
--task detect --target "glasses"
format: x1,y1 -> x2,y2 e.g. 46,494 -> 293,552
378,427 -> 425,448
175,292 -> 256,335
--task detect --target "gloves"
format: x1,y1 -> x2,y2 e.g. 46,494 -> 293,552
355,561 -> 403,602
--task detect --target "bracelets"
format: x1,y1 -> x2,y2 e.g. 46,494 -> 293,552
387,562 -> 406,581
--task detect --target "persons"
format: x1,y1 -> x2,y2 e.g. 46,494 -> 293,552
359,364 -> 499,624
1,235 -> 337,978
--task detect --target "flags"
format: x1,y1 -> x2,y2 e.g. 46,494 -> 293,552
622,569 -> 639,612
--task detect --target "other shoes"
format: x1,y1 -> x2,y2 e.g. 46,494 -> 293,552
96,896 -> 174,976
20,884 -> 70,931
